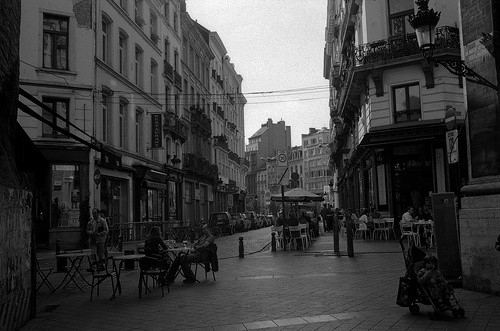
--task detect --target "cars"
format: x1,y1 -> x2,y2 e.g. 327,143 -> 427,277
209,212 -> 275,235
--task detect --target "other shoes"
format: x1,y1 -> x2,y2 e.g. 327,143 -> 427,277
439,302 -> 451,310
87,268 -> 96,272
98,267 -> 105,271
183,279 -> 195,284
163,279 -> 174,283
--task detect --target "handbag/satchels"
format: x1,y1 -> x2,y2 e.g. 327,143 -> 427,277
396,275 -> 416,307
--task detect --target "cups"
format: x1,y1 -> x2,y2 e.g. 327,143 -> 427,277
419,220 -> 423,222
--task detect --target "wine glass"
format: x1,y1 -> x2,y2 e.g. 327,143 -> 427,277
182,241 -> 188,249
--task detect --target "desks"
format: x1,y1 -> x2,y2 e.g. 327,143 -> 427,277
165,246 -> 194,282
413,220 -> 433,247
54,252 -> 92,295
110,253 -> 149,300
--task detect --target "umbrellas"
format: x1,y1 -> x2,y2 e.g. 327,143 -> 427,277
271,188 -> 325,202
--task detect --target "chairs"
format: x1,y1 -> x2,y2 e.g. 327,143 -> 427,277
137,256 -> 170,298
193,243 -> 217,284
35,255 -> 54,294
271,216 -> 434,254
87,253 -> 122,302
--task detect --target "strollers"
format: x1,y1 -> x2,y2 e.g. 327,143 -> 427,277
399,233 -> 468,321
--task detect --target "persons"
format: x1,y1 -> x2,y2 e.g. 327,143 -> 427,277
143,226 -> 170,272
417,255 -> 455,299
86,208 -> 111,272
401,204 -> 433,247
162,223 -> 215,285
276,211 -> 319,251
320,204 -> 381,240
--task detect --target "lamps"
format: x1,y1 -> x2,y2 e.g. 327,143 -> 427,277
406,0 -> 500,104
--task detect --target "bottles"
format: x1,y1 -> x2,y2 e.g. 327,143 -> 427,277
174,243 -> 178,248
